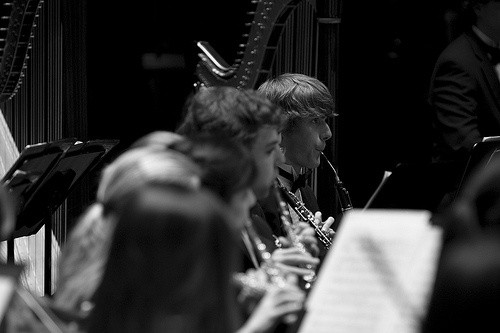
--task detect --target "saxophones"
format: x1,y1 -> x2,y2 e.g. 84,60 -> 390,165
274,176 -> 334,251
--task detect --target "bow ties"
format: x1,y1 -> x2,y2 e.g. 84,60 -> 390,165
277,167 -> 307,195
465,25 -> 500,67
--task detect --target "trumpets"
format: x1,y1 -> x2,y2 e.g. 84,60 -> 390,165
319,151 -> 353,213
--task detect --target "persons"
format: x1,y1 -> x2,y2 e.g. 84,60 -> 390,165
425,0 -> 500,209
54,72 -> 334,333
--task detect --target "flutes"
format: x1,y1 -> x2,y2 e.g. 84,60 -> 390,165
245,220 -> 297,324
